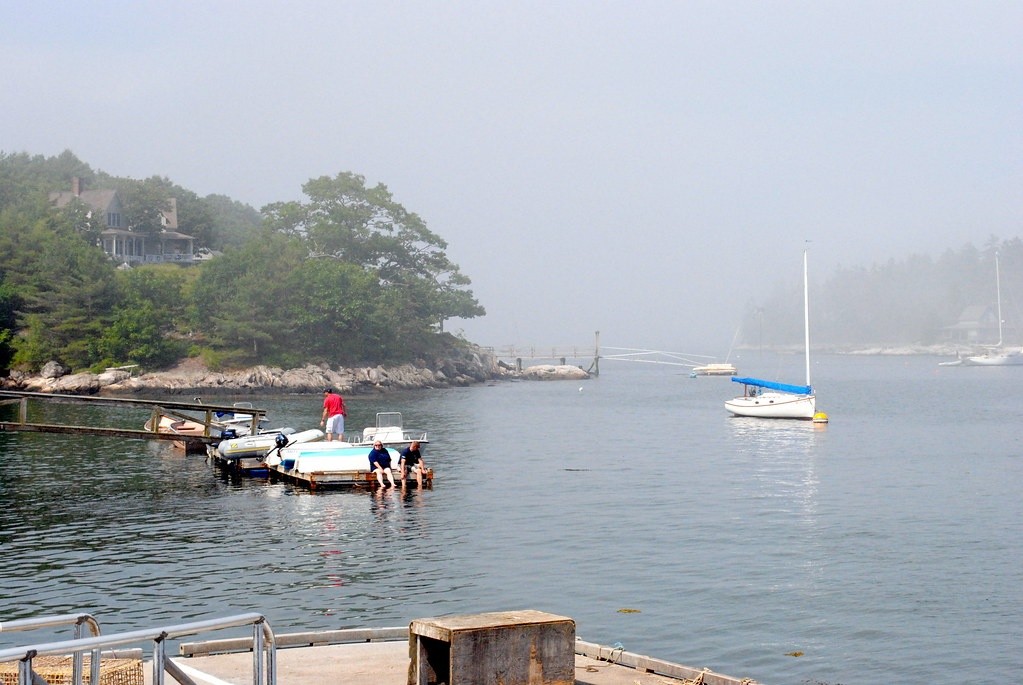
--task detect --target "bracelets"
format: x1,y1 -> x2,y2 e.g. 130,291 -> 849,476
321,419 -> 324,421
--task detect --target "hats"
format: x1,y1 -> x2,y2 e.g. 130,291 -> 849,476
323,387 -> 332,393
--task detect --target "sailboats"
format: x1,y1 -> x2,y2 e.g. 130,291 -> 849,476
693,325 -> 737,374
725,248 -> 817,420
963,250 -> 1022,365
939,350 -> 963,366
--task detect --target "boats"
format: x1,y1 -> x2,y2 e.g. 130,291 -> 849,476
193,397 -> 270,428
346,412 -> 430,458
219,425 -> 324,458
145,407 -> 207,450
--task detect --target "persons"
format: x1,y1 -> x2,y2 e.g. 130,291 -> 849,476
320,386 -> 347,442
368,440 -> 397,489
397,441 -> 426,491
